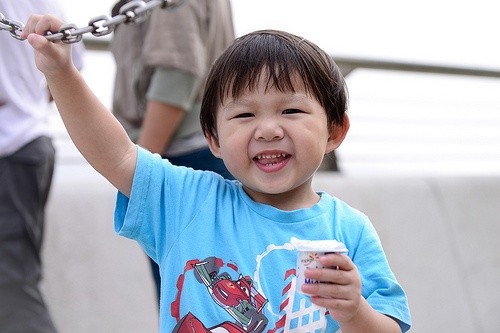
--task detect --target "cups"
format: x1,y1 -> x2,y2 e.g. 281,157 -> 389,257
291,238 -> 349,297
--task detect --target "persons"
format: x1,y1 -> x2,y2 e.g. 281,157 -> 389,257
1,0 -> 85,333
21,16 -> 411,333
108,1 -> 238,322
